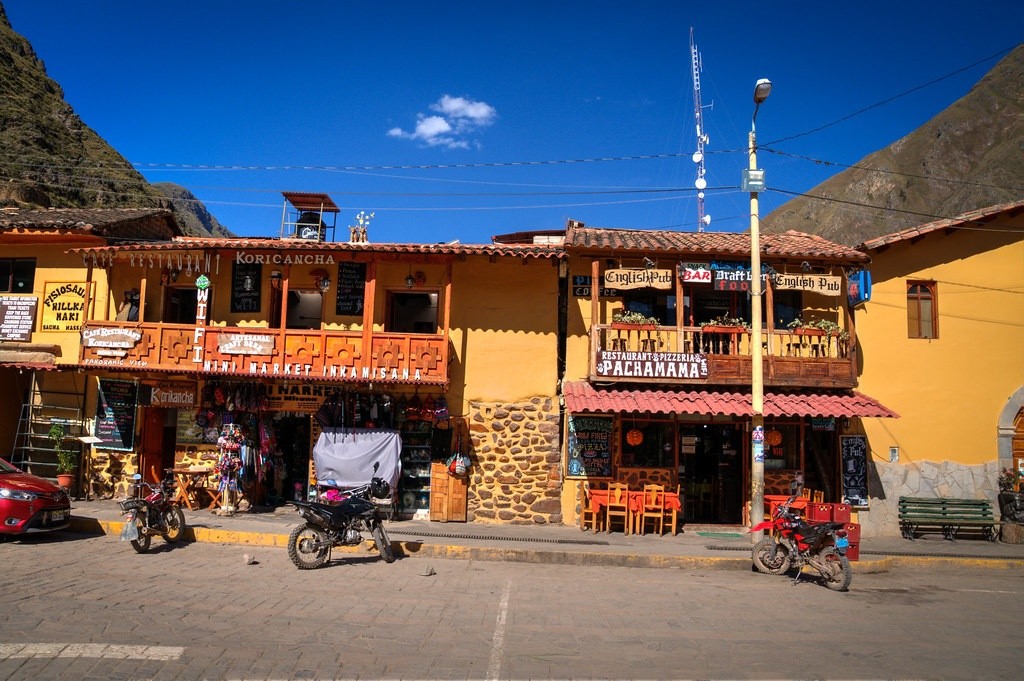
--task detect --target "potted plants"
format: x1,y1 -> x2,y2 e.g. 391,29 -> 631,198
611,312 -> 849,339
50,422 -> 75,487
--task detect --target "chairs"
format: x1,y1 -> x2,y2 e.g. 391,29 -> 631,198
779,343 -> 826,358
799,488 -> 825,518
208,477 -> 224,508
581,480 -> 680,536
152,465 -> 177,505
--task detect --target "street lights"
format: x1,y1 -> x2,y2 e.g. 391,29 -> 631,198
742,78 -> 774,554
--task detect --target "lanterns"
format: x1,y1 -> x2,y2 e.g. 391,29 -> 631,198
765,430 -> 781,445
627,429 -> 643,446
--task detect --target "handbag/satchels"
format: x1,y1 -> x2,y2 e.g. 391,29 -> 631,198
312,384 -> 471,478
195,381 -> 285,515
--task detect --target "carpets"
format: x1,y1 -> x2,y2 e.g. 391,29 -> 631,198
697,532 -> 743,536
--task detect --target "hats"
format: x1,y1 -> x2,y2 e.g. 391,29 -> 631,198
295,483 -> 303,490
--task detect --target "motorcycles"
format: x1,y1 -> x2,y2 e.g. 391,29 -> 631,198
286,461 -> 395,571
116,467 -> 186,554
747,478 -> 852,591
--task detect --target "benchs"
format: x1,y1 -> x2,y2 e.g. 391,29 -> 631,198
899,496 -> 1004,542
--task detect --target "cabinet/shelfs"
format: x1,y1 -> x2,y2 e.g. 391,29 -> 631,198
402,429 -> 429,513
11,404 -> 83,499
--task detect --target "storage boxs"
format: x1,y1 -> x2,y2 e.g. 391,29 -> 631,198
808,503 -> 861,561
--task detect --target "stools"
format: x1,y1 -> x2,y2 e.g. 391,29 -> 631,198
612,338 -> 659,352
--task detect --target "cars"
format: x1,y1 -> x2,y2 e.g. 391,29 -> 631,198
0,457 -> 71,542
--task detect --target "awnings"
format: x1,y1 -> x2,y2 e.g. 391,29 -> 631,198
563,381 -> 901,418
0,349 -> 57,371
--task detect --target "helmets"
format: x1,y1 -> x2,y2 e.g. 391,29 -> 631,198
371,477 -> 390,499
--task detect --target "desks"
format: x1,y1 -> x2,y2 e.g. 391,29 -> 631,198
589,489 -> 682,536
764,495 -> 810,539
164,467 -> 211,510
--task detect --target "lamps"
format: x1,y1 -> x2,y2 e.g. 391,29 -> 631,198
243,274 -> 253,291
322,264 -> 331,288
172,267 -> 178,282
406,264 -> 414,289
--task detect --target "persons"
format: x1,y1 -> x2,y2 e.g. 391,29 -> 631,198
116,288 -> 149,321
